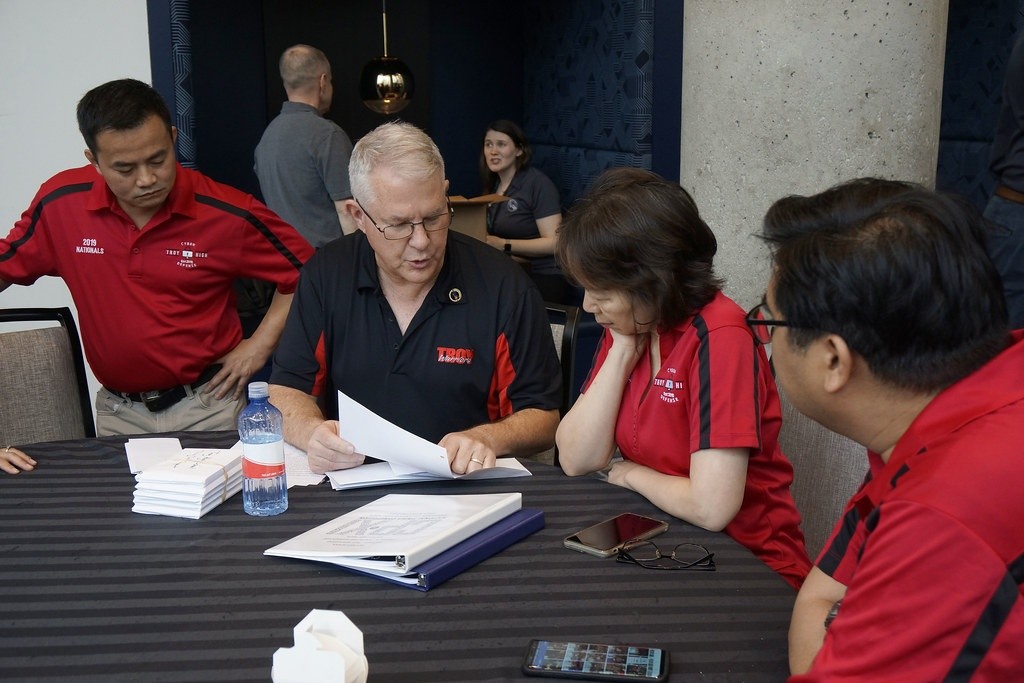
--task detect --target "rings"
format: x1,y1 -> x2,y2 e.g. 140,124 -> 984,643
6,444 -> 12,451
471,458 -> 483,464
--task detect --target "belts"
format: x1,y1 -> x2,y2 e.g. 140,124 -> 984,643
104,363 -> 224,412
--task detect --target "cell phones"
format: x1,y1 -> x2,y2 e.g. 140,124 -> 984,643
520,639 -> 670,683
563,513 -> 668,560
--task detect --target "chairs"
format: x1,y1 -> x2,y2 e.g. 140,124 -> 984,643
1,308 -> 97,444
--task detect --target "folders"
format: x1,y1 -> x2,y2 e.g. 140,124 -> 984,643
263,492 -> 522,574
326,508 -> 545,593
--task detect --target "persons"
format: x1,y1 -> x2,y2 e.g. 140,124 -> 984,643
551,158 -> 817,596
760,175 -> 1024,683
260,109 -> 563,478
253,45 -> 360,251
0,71 -> 314,485
980,28 -> 1024,331
476,118 -> 562,267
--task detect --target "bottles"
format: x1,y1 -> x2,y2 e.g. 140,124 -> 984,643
238,381 -> 289,517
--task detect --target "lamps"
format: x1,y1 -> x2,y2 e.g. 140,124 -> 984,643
363,0 -> 412,114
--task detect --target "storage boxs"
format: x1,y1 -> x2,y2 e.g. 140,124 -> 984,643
446,194 -> 511,246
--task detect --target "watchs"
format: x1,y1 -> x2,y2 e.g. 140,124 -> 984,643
504,239 -> 511,254
823,596 -> 845,628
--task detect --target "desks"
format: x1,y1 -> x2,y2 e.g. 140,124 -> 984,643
0,430 -> 800,682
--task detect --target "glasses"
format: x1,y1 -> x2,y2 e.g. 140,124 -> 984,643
744,302 -> 854,352
356,188 -> 455,240
617,540 -> 716,572
